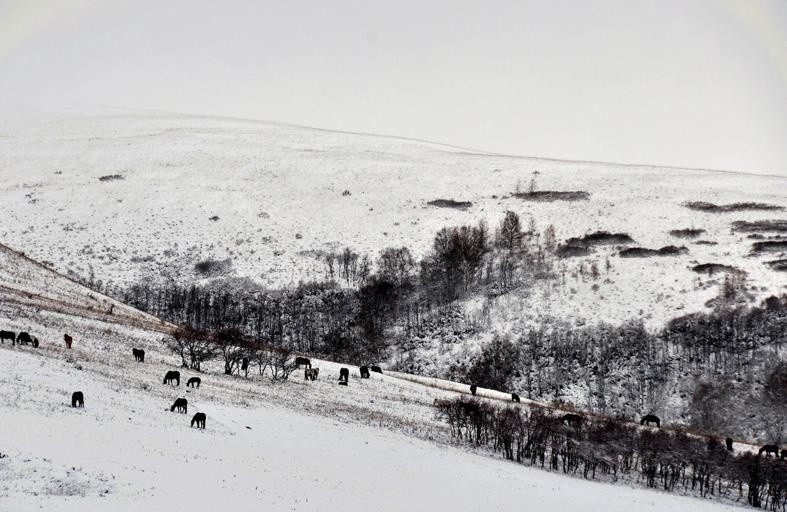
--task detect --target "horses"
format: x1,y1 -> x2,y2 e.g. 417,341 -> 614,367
559,414 -> 584,426
510,392 -> 523,401
725,436 -> 787,462
160,368 -> 210,430
1,328 -> 41,349
294,355 -> 386,386
63,332 -> 75,348
70,389 -> 90,409
640,413 -> 662,429
469,383 -> 479,397
131,348 -> 146,363
